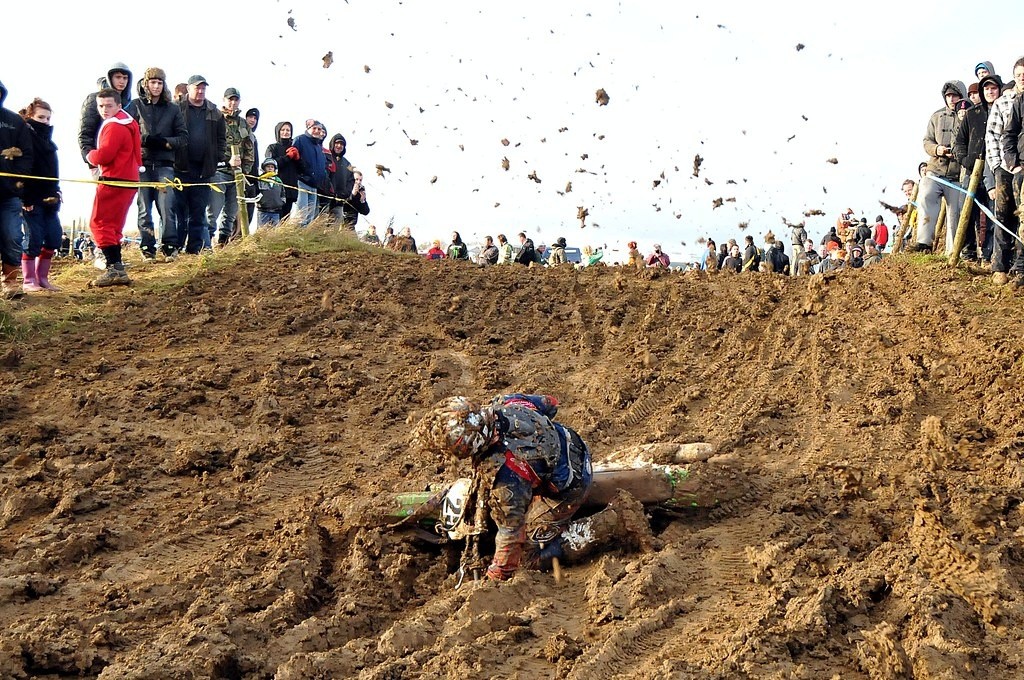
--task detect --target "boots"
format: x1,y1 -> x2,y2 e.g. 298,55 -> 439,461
561,488 -> 653,560
1,261 -> 25,295
20,257 -> 62,292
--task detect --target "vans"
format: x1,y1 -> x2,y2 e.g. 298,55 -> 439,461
542,247 -> 581,264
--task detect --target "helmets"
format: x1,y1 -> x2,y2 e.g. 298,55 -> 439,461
429,395 -> 495,458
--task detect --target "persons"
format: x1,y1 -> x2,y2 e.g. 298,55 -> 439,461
791,220 -> 807,278
84,88 -> 141,285
382,227 -> 417,254
265,122 -> 308,222
204,87 -> 254,249
628,241 -> 646,268
699,229 -> 790,275
52,232 -> 96,260
426,240 -> 446,259
417,394 -> 653,602
646,244 -> 670,268
672,261 -> 699,273
871,213 -> 889,254
892,57 -> 1024,286
360,225 -> 382,249
256,157 -> 287,232
0,81 -> 33,297
123,67 -> 227,263
584,246 -> 603,266
292,119 -> 370,242
77,61 -> 136,270
446,231 -> 469,261
477,233 -> 584,265
794,209 -> 882,274
17,101 -> 63,292
124,237 -> 128,247
230,107 -> 260,242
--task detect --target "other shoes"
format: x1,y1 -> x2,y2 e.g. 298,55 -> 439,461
91,262 -> 131,286
141,248 -> 157,263
992,271 -> 1008,284
162,244 -> 179,262
909,242 -> 933,255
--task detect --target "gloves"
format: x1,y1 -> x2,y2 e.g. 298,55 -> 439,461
285,147 -> 300,161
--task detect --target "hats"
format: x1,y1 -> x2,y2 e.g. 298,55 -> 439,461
852,248 -> 862,253
433,240 -> 440,246
967,83 -> 980,98
982,79 -> 998,87
956,101 -> 972,112
306,119 -> 322,130
861,218 -> 866,223
876,215 -> 883,222
263,159 -> 277,170
247,109 -> 259,119
143,67 -> 172,104
335,139 -> 345,144
944,87 -> 959,96
974,64 -> 988,74
188,75 -> 210,87
831,227 -> 836,232
558,237 -> 566,247
223,88 -> 240,99
828,241 -> 838,250
